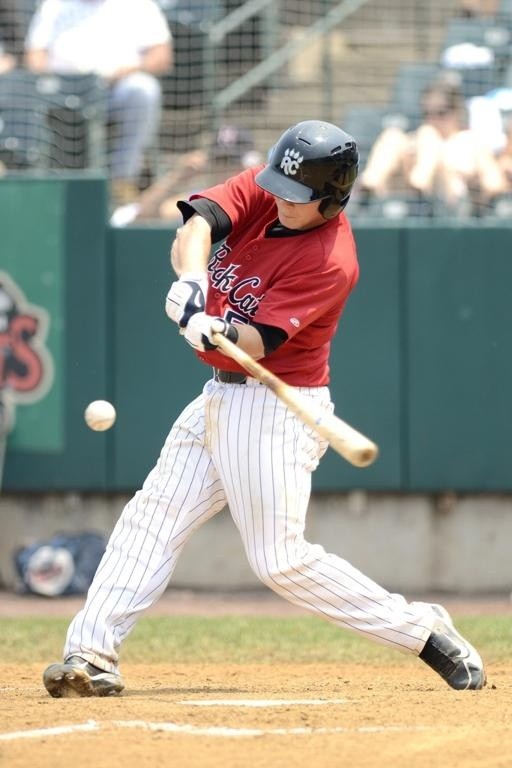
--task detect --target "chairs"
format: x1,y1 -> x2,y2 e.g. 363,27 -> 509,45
0,0 -> 512,217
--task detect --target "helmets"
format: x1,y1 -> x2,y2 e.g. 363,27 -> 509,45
253,119 -> 359,220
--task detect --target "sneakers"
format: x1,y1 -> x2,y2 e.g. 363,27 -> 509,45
43,656 -> 126,699
417,602 -> 487,691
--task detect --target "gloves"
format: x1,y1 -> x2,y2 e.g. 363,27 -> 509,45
164,279 -> 208,337
182,311 -> 238,352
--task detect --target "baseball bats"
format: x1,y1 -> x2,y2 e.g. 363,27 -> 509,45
211,332 -> 379,468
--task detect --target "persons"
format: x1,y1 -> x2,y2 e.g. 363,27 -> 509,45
348,69 -> 512,219
36,115 -> 487,703
109,124 -> 266,228
21,0 -> 179,217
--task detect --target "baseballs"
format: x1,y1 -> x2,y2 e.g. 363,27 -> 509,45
84,398 -> 116,431
